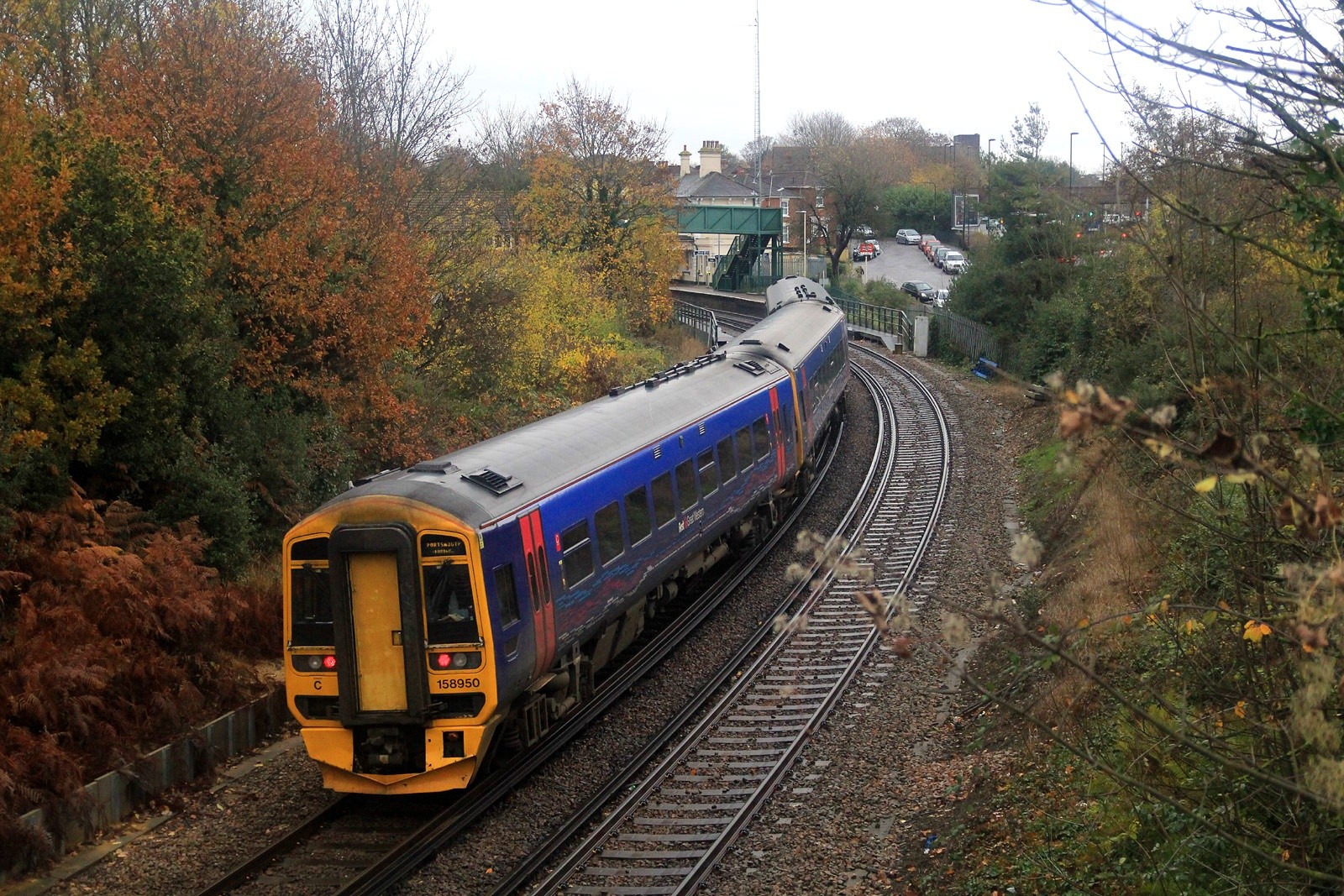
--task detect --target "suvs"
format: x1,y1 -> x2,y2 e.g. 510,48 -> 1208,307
942,250 -> 966,274
931,289 -> 951,309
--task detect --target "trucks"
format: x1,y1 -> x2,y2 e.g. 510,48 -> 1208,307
1104,215 -> 1130,224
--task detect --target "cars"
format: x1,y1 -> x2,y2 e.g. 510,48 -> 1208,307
895,228 -> 922,245
866,240 -> 880,254
901,280 -> 936,302
924,240 -> 942,256
918,234 -> 937,249
934,247 -> 952,267
988,220 -> 1004,239
928,245 -> 944,262
848,225 -> 875,239
853,243 -> 877,261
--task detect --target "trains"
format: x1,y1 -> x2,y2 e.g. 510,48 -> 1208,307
281,274 -> 852,798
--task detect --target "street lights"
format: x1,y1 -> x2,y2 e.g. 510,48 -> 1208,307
1101,142 -> 1105,185
795,210 -> 807,279
1070,132 -> 1079,193
944,142 -> 972,248
988,139 -> 997,234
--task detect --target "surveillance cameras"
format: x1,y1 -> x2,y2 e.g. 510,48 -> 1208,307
777,188 -> 784,192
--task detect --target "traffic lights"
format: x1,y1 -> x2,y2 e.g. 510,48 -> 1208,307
1089,211 -> 1093,216
